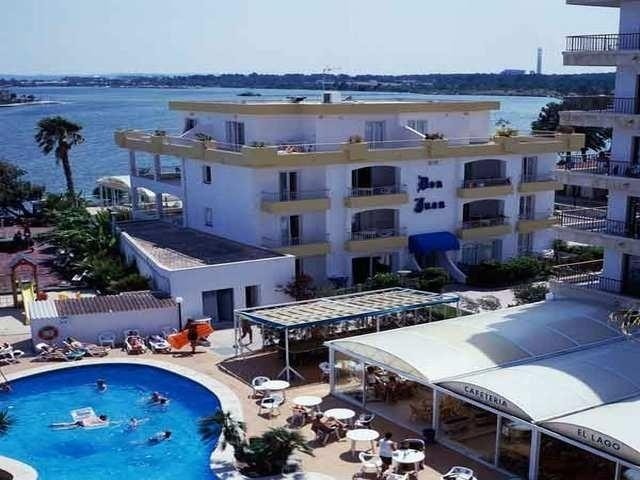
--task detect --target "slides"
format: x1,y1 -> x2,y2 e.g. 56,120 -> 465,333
21,280 -> 35,325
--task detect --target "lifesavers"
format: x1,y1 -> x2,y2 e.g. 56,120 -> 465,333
38,326 -> 58,340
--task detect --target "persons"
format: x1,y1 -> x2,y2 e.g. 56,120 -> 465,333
48,414 -> 107,431
376,432 -> 398,476
97,379 -> 107,393
128,336 -> 144,353
68,336 -> 96,357
152,392 -> 170,404
179,318 -> 206,353
161,431 -> 171,440
596,151 -> 609,174
365,367 -> 396,402
317,412 -> 349,440
131,418 -> 140,429
0,342 -> 9,353
238,318 -> 253,344
260,308 -> 436,350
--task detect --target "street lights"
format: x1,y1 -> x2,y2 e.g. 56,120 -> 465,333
175,296 -> 183,333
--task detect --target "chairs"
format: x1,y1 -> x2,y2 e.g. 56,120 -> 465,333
0,324 -> 181,365
255,374 -> 476,480
